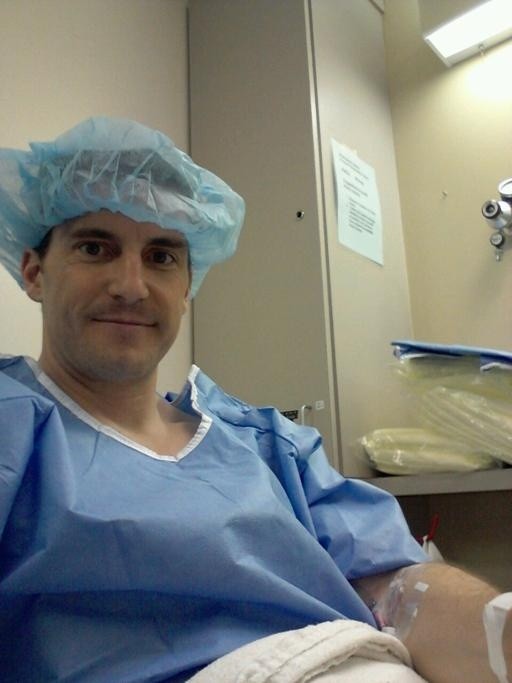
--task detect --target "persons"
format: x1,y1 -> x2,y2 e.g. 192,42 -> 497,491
0,117 -> 512,683
